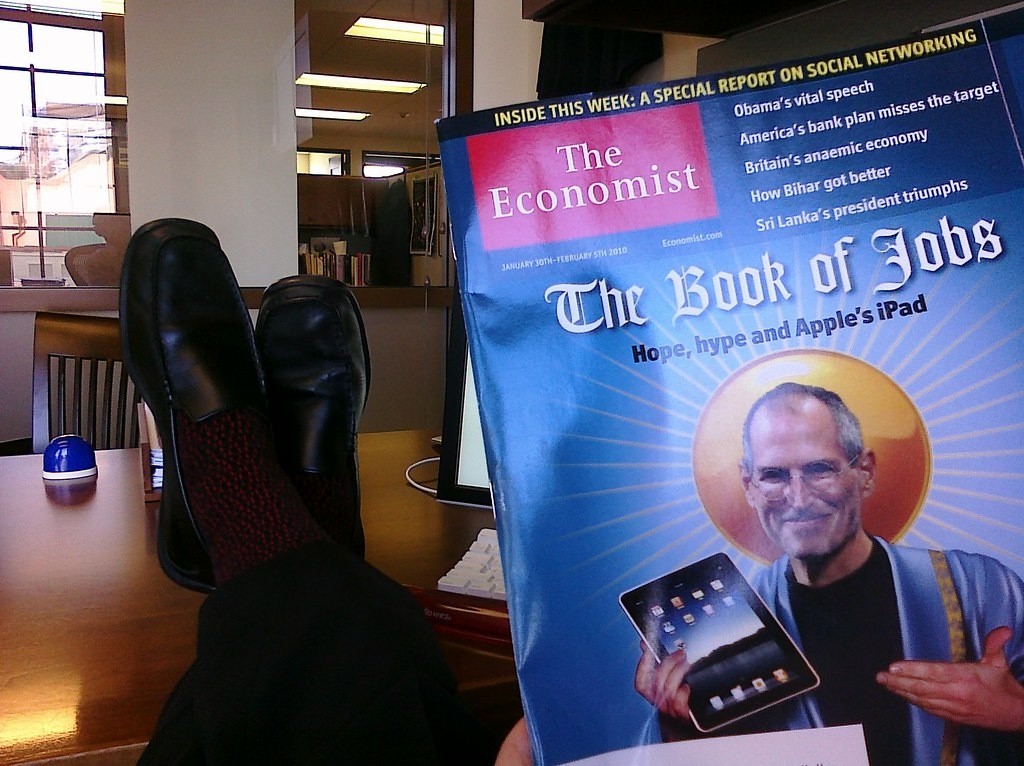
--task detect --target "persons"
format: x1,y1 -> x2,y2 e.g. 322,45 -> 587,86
119,218 -> 531,766
636,382 -> 1023,765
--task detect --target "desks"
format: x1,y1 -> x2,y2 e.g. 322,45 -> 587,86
0,426 -> 528,764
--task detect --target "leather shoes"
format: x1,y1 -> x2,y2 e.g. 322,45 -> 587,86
255,274 -> 373,560
120,217 -> 263,594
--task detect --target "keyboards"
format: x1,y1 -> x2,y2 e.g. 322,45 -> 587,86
438,527 -> 509,604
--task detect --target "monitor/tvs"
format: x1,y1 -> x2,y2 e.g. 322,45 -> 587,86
438,259 -> 496,511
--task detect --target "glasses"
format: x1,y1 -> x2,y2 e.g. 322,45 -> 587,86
746,454 -> 859,502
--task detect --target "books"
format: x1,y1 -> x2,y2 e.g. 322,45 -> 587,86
298,250 -> 371,288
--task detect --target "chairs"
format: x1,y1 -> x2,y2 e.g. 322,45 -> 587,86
30,309 -> 147,453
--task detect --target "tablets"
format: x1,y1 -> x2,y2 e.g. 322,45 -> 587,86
619,553 -> 820,734
